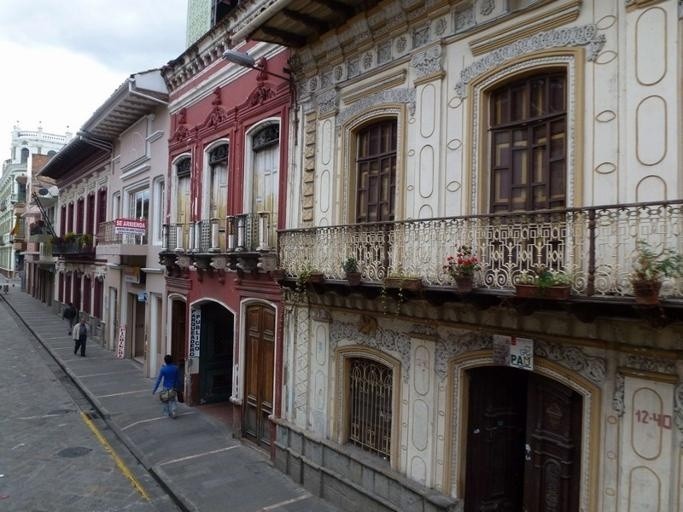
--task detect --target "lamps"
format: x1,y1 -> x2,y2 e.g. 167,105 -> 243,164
219,50 -> 299,147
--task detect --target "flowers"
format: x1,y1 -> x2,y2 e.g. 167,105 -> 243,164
441,242 -> 482,281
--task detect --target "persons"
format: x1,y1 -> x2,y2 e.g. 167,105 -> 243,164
72,317 -> 90,357
152,355 -> 179,420
62,303 -> 76,335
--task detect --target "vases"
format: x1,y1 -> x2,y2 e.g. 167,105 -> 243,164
453,275 -> 473,293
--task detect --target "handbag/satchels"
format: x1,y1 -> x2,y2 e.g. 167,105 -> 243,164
160,389 -> 175,402
178,391 -> 183,403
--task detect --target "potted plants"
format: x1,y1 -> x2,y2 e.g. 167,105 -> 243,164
288,269 -> 324,312
340,254 -> 361,286
515,265 -> 574,302
379,272 -> 422,316
628,235 -> 683,306
